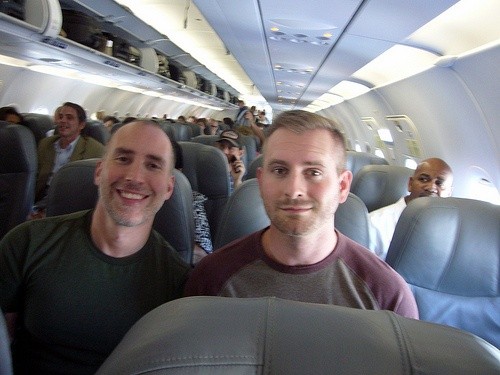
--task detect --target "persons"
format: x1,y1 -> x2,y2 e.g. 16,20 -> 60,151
185,109 -> 419,320
0,122 -> 193,375
368,157 -> 454,262
0,100 -> 279,258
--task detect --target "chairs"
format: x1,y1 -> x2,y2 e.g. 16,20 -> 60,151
0,112 -> 500,375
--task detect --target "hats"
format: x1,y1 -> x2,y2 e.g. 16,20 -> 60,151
216,130 -> 241,147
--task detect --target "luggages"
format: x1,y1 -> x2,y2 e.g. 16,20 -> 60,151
61,8 -> 102,50
103,30 -> 139,68
158,55 -> 170,77
171,65 -> 186,84
197,74 -> 225,100
